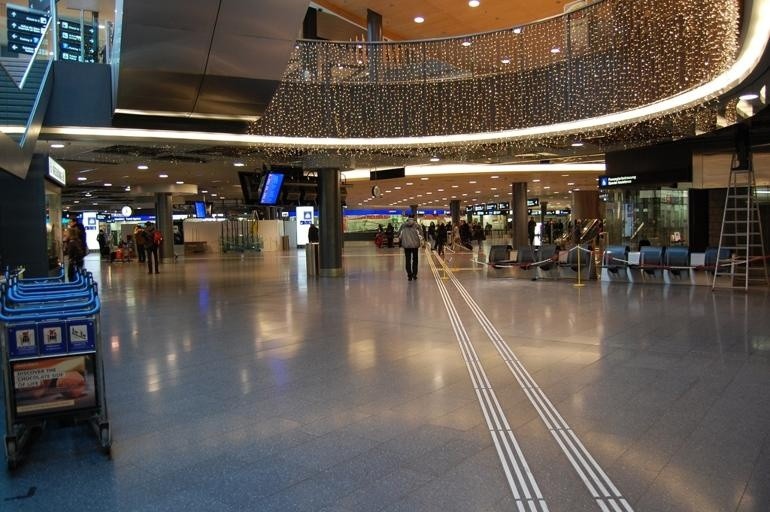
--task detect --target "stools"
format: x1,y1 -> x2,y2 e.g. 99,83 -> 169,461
183,242 -> 207,255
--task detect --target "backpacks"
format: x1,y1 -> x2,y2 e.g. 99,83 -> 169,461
154,227 -> 161,246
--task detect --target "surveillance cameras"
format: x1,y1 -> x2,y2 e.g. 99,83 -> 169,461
318,9 -> 323,12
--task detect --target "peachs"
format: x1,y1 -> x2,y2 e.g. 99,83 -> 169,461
57,371 -> 84,398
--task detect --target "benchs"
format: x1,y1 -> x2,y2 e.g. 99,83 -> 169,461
219,234 -> 264,252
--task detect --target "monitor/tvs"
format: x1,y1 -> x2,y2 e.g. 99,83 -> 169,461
259,169 -> 287,205
237,170 -> 261,205
194,200 -> 206,218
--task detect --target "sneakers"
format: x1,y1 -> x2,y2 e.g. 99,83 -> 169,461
406,275 -> 418,281
137,259 -> 160,275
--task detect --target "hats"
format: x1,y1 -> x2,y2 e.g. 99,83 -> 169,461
136,222 -> 154,228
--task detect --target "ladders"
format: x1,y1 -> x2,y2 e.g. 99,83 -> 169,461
711,148 -> 770,292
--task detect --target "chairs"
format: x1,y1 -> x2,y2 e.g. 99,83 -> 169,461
487,246 -> 732,283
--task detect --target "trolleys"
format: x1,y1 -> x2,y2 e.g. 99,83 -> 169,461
0,264 -> 113,473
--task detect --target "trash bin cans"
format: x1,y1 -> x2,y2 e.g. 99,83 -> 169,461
306,242 -> 320,276
283,235 -> 290,250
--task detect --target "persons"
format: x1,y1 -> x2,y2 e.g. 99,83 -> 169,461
133,225 -> 146,264
146,274 -> 161,317
96,229 -> 106,257
107,234 -> 137,265
399,281 -> 422,341
374,215 -> 604,256
637,234 -> 652,254
141,221 -> 160,274
396,212 -> 423,281
308,225 -> 319,243
62,216 -> 88,283
